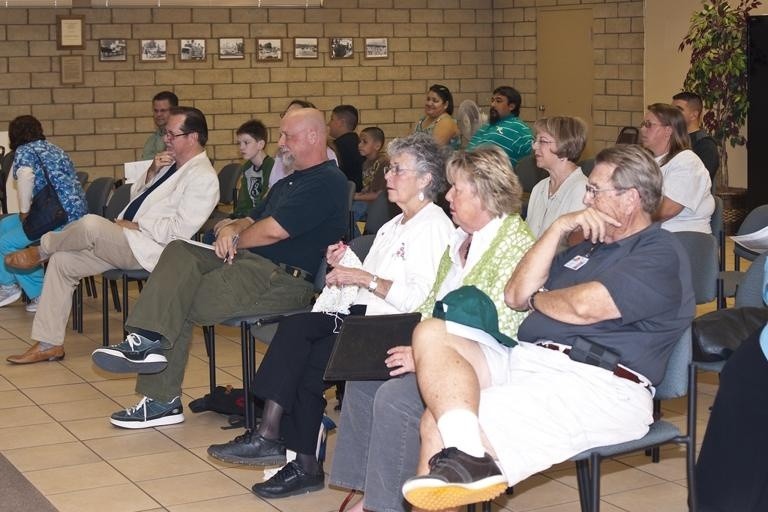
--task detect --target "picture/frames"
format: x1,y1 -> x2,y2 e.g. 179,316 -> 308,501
177,36 -> 209,63
293,36 -> 319,63
256,37 -> 283,63
139,37 -> 169,64
364,34 -> 389,61
216,35 -> 246,62
96,36 -> 127,63
56,14 -> 86,51
329,35 -> 354,60
60,54 -> 85,87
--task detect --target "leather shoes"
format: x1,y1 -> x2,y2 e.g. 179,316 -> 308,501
207,426 -> 287,466
4,246 -> 43,270
7,341 -> 65,364
252,460 -> 324,498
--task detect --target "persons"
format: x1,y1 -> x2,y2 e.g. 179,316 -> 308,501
690,259 -> 768,512
4,106 -> 221,365
327,145 -> 537,512
206,132 -> 454,498
141,90 -> 181,163
641,92 -> 719,236
327,84 -> 458,230
197,97 -> 339,242
0,115 -> 88,312
471,85 -> 591,244
91,107 -> 347,429
401,143 -> 693,512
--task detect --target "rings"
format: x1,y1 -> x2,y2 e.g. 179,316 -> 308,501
160,157 -> 163,161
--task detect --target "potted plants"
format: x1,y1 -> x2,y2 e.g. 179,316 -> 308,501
668,0 -> 762,236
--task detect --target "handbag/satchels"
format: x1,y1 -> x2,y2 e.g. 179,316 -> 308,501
692,306 -> 767,363
15,144 -> 68,241
263,411 -> 337,481
188,385 -> 265,418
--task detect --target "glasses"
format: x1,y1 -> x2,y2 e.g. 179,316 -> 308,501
244,141 -> 257,144
586,182 -> 615,199
530,136 -> 558,146
384,163 -> 416,176
154,108 -> 168,113
160,127 -> 192,140
639,120 -> 672,128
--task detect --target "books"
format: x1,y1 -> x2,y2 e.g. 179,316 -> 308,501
322,310 -> 422,380
727,226 -> 767,254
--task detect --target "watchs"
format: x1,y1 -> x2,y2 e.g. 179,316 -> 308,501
368,272 -> 380,293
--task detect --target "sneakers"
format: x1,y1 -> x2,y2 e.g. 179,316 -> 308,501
110,395 -> 185,429
91,333 -> 168,375
0,283 -> 22,308
401,447 -> 508,511
25,295 -> 41,312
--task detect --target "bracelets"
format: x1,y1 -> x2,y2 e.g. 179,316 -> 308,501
527,287 -> 551,311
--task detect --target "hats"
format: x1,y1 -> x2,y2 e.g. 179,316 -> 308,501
432,285 -> 518,348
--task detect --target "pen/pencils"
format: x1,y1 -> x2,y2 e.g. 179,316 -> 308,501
223,234 -> 239,263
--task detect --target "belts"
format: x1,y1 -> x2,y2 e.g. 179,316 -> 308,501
272,259 -> 316,284
536,342 -> 652,395
